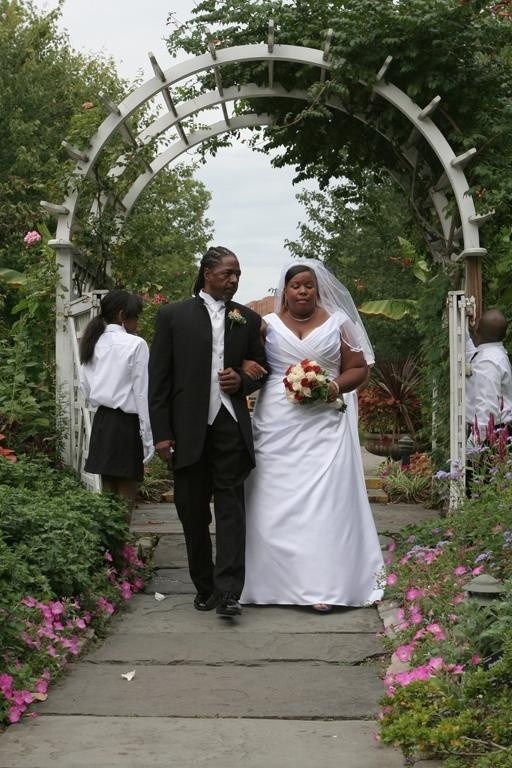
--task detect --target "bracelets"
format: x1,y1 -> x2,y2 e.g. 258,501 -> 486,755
330,380 -> 339,395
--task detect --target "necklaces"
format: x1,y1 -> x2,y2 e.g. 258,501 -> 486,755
286,308 -> 319,322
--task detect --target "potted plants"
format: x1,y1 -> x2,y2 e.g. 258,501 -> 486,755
358,350 -> 433,460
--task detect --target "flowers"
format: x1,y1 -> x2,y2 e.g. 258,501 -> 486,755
282,358 -> 348,412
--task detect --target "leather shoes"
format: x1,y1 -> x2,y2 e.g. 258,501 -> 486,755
194,589 -> 219,611
217,592 -> 243,616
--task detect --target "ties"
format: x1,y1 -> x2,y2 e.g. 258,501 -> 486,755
208,303 -> 222,318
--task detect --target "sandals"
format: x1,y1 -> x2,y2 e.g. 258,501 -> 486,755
314,603 -> 331,611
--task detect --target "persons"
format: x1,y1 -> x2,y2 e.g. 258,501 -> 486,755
147,244 -> 274,619
79,288 -> 157,533
237,254 -> 391,613
463,306 -> 512,504
